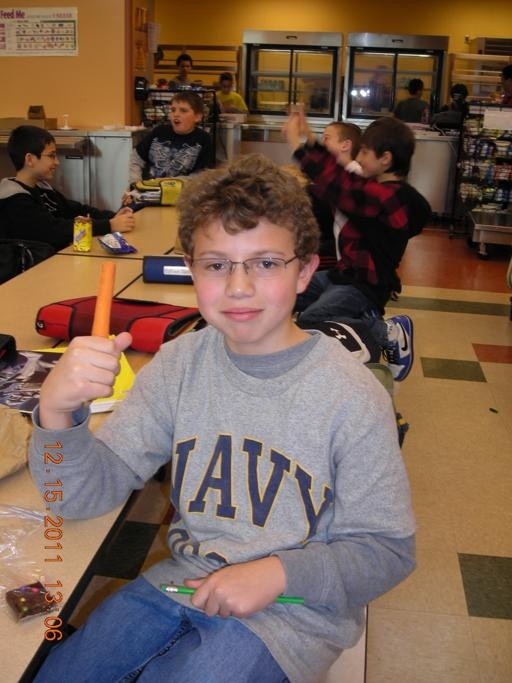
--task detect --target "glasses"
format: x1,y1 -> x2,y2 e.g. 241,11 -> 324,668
183,250 -> 304,280
28,151 -> 58,160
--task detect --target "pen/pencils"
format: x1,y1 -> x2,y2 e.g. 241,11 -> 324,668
161,583 -> 305,606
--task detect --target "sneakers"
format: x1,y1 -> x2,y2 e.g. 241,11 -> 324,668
378,314 -> 415,382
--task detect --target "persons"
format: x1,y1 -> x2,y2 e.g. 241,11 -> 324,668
500,66 -> 511,109
169,53 -> 197,88
27,152 -> 421,683
437,84 -> 468,129
216,72 -> 250,113
154,46 -> 164,65
1,124 -> 136,286
282,97 -> 431,382
118,90 -> 215,214
395,79 -> 427,122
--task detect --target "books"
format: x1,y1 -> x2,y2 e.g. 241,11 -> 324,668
459,118 -> 511,213
